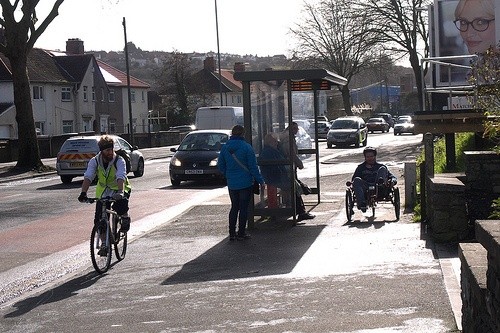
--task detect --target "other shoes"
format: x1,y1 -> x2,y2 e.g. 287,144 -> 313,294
121,216 -> 130,231
237,232 -> 253,240
378,177 -> 383,185
361,202 -> 366,212
98,245 -> 107,255
271,216 -> 276,222
230,234 -> 238,240
298,213 -> 314,219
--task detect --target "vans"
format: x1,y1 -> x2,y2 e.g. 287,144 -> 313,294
195,106 -> 258,143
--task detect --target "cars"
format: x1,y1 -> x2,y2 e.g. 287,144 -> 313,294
272,113 -> 415,157
168,129 -> 231,187
56,134 -> 144,184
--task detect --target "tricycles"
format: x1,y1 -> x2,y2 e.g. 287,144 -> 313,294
344,176 -> 401,222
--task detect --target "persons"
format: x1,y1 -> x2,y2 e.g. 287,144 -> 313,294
281,122 -> 303,207
217,125 -> 265,240
259,133 -> 316,219
352,147 -> 397,212
453,0 -> 495,54
78,136 -> 131,257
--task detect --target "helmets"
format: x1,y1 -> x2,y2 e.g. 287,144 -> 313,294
364,147 -> 376,156
98,137 -> 113,150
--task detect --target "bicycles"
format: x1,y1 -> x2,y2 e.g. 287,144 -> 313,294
83,195 -> 129,274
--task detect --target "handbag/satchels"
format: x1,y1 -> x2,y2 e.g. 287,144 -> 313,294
298,180 -> 311,195
253,181 -> 259,195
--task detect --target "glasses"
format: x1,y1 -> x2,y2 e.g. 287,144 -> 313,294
453,17 -> 495,32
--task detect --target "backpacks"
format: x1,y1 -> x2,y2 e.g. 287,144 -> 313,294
96,150 -> 130,174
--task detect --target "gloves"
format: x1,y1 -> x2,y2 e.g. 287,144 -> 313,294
78,192 -> 87,202
115,192 -> 123,200
